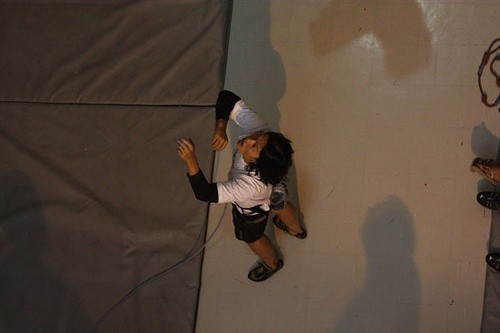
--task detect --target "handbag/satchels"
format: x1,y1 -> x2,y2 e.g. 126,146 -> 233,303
270,182 -> 288,210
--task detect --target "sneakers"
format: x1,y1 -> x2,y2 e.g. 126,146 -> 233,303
272,216 -> 307,239
248,258 -> 284,282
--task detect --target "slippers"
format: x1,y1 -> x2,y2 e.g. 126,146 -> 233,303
472,158 -> 500,185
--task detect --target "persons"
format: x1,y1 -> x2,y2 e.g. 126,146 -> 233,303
177,90 -> 307,282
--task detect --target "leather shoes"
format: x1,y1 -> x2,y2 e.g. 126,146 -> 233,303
477,192 -> 500,211
486,252 -> 500,272
